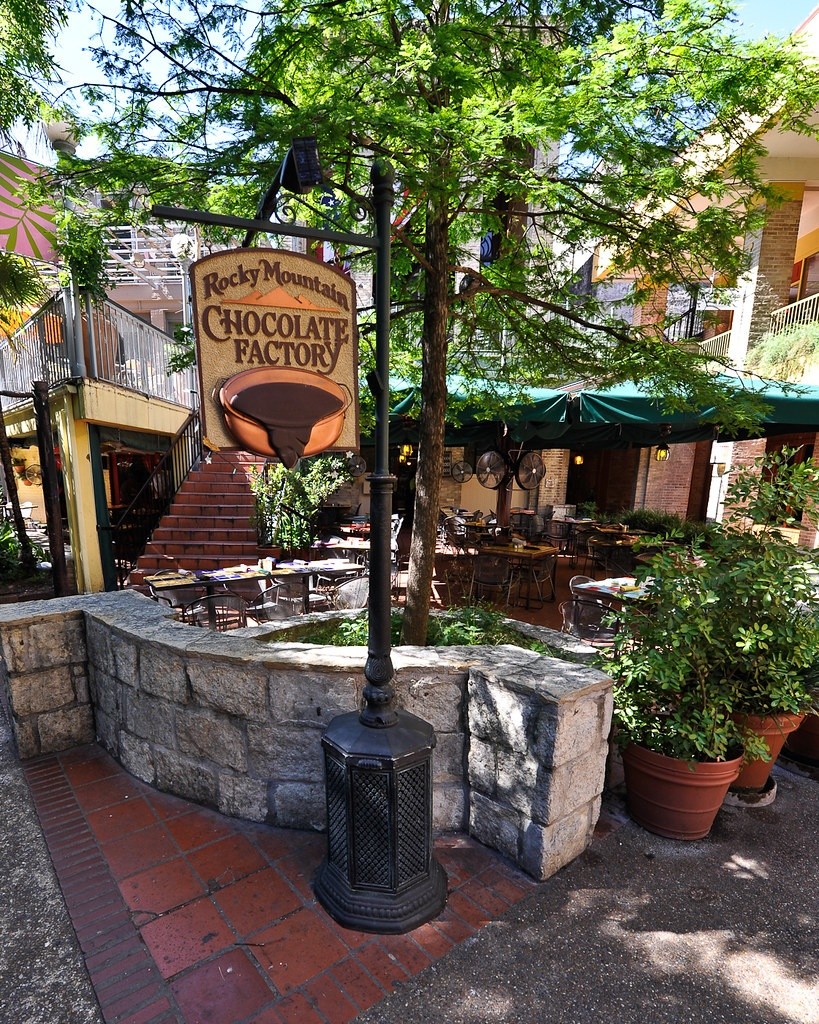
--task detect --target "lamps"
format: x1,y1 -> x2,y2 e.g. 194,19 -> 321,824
654,444 -> 670,460
710,462 -> 726,477
398,444 -> 417,465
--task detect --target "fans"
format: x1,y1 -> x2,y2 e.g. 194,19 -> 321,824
346,456 -> 366,478
451,461 -> 473,483
475,449 -> 509,490
26,464 -> 42,485
513,450 -> 545,491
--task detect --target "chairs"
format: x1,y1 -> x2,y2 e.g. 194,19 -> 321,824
2,501 -> 37,532
140,501 -> 681,651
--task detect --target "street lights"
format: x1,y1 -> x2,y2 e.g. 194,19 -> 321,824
278,132 -> 450,938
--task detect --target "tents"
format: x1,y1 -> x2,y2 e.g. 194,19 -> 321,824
353,366 -> 819,449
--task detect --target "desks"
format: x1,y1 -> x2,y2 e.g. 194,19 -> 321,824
591,525 -> 653,535
480,542 -> 560,610
261,563 -> 365,615
324,539 -> 396,565
548,518 -> 597,555
337,526 -> 370,540
572,576 -> 657,613
4,504 -> 39,509
143,566 -> 267,632
510,510 -> 538,541
459,520 -> 496,534
588,538 -> 671,579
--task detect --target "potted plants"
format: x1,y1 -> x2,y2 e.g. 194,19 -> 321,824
596,442 -> 819,840
707,315 -> 727,332
247,454 -> 356,568
12,457 -> 33,486
48,212 -> 118,380
